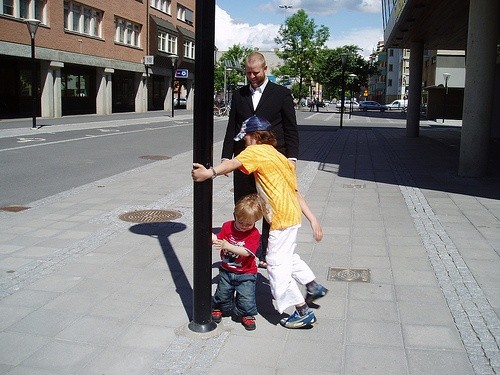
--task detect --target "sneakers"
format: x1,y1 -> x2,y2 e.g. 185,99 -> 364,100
212,306 -> 221,322
281,311 -> 317,328
305,285 -> 328,305
241,315 -> 256,330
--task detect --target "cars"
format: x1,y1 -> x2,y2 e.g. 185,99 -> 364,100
299,98 -> 330,108
173,98 -> 186,107
336,101 -> 359,110
359,101 -> 388,112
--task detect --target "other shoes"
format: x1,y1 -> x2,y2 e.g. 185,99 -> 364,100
257,258 -> 268,269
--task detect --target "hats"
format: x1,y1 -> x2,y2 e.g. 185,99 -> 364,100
234,115 -> 271,141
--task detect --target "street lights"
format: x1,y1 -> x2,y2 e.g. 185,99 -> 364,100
24,18 -> 42,129
443,73 -> 451,123
348,74 -> 357,119
226,68 -> 232,103
168,55 -> 179,118
340,53 -> 349,127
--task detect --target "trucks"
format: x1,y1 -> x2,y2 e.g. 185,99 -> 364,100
385,100 -> 408,109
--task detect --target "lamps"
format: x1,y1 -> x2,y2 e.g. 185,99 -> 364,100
170,81 -> 173,88
106,74 -> 109,82
143,78 -> 146,84
52,69 -> 57,79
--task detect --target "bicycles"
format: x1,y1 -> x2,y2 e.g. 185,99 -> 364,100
214,105 -> 231,118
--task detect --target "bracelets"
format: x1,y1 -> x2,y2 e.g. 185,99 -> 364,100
210,168 -> 216,178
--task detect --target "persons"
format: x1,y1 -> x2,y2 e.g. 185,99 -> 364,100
221,52 -> 299,269
191,115 -> 327,328
212,194 -> 263,330
307,98 -> 319,112
